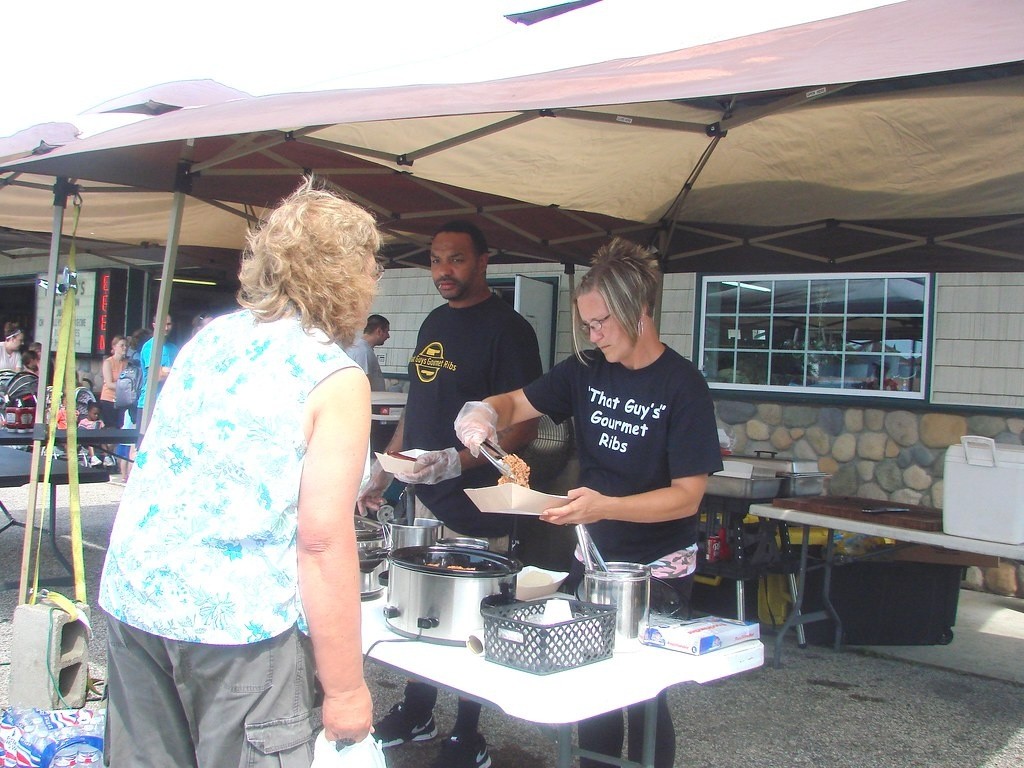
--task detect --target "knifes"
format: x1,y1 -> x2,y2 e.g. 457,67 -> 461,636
863,506 -> 910,513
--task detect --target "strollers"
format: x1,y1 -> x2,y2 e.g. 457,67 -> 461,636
40,386 -> 87,468
75,378 -> 116,476
0,369 -> 39,452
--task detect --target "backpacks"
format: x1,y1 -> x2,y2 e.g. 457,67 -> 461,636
115,359 -> 143,410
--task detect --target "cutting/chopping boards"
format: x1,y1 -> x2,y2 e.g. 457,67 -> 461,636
773,495 -> 942,532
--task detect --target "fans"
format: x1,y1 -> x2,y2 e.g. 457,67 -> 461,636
506,415 -> 576,560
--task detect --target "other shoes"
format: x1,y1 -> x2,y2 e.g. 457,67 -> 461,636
77,445 -> 82,454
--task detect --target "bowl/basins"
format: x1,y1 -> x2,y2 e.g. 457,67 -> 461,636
515,565 -> 569,601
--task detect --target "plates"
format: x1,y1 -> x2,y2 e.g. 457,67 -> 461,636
373,448 -> 432,474
463,483 -> 575,516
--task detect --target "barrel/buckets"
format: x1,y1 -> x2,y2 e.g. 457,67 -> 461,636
386,517 -> 444,569
584,561 -> 651,639
434,537 -> 489,552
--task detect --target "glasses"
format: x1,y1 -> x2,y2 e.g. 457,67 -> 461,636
197,313 -> 207,326
370,264 -> 385,281
581,314 -> 610,333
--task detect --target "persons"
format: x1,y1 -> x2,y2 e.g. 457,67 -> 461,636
347,315 -> 390,392
454,237 -> 723,767
357,221 -> 543,768
98,172 -> 385,767
0,311 -> 214,482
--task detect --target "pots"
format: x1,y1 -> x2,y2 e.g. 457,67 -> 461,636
382,546 -> 522,648
353,514 -> 391,602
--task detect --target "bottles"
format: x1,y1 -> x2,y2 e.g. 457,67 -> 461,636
0,708 -> 105,768
5,407 -> 36,433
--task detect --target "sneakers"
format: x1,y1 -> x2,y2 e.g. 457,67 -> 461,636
371,702 -> 438,748
90,456 -> 114,466
432,734 -> 491,768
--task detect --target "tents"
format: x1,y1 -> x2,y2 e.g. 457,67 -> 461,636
0,0 -> 1024,607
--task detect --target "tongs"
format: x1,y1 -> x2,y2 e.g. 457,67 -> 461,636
574,522 -> 609,571
479,438 -> 522,481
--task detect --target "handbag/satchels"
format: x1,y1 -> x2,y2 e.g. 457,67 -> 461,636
311,728 -> 387,768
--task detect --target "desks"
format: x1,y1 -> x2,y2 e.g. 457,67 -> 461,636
346,565 -> 763,768
750,502 -> 1024,670
0,428 -> 140,589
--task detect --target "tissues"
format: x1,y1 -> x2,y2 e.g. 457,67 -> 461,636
522,599 -> 605,667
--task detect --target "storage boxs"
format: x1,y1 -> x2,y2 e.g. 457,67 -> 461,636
643,435 -> 1024,655
786,542 -> 969,649
